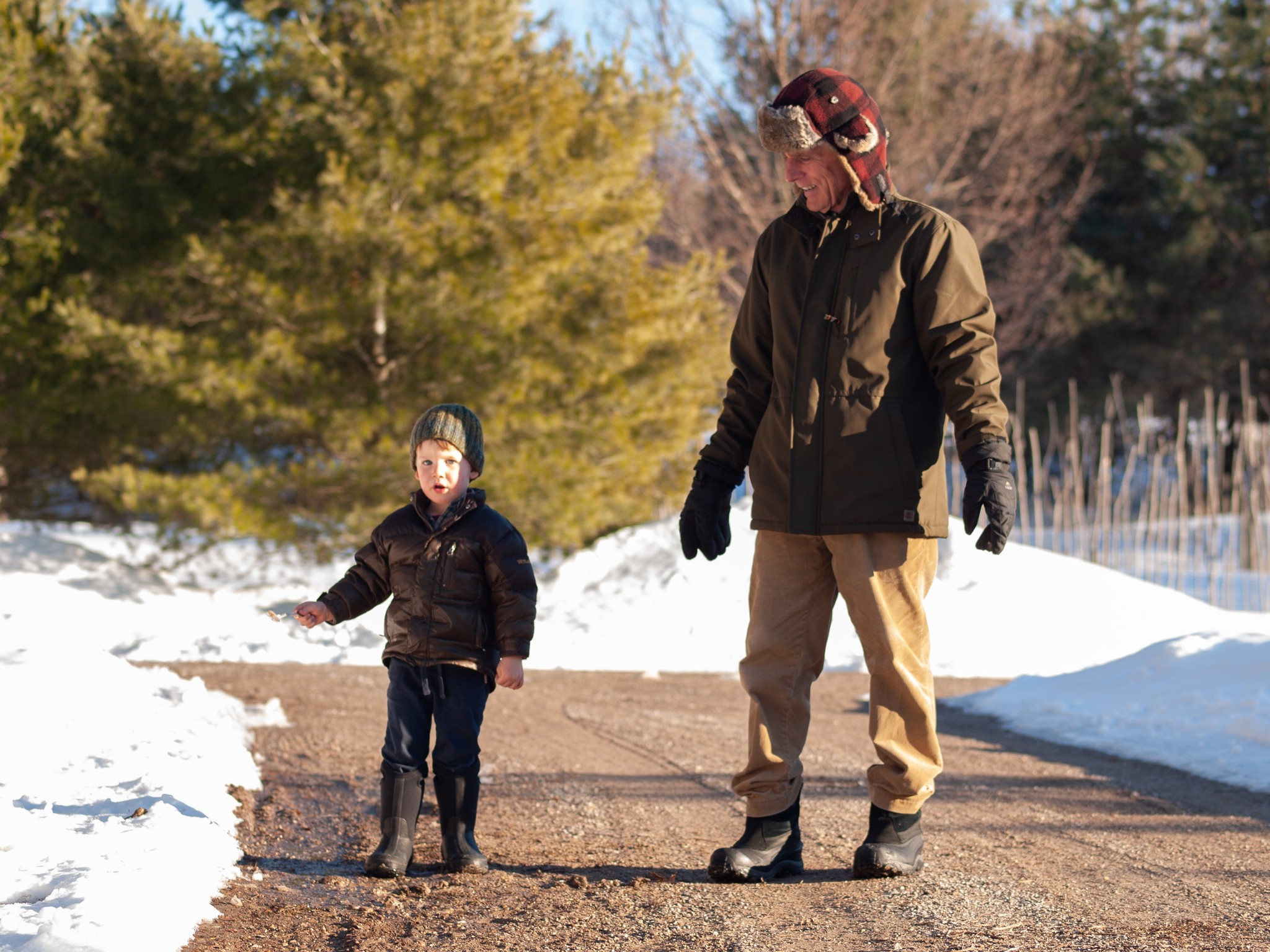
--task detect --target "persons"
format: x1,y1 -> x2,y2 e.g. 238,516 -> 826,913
679,67 -> 1016,877
295,404 -> 539,878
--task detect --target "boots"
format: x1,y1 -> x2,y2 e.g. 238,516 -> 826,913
432,773 -> 489,874
853,800 -> 924,877
707,783 -> 805,883
363,761 -> 429,877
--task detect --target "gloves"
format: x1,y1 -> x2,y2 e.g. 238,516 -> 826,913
679,458 -> 745,560
962,439 -> 1017,554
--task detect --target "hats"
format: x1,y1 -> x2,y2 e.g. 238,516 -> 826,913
410,404 -> 485,482
757,67 -> 896,212
